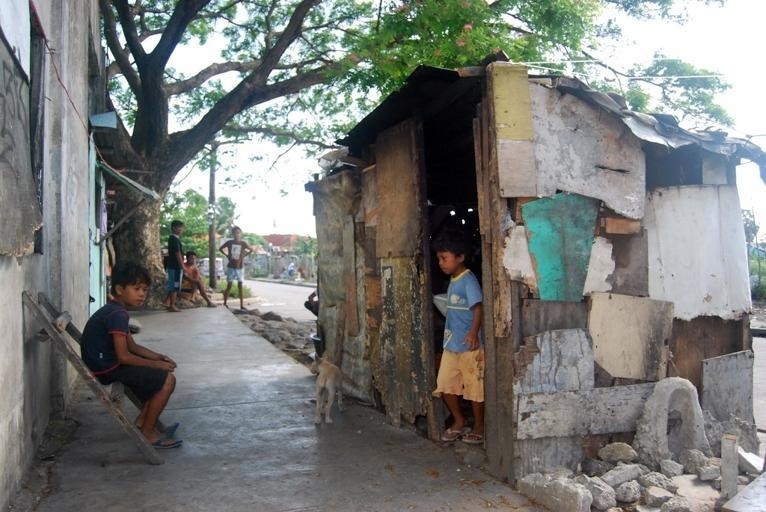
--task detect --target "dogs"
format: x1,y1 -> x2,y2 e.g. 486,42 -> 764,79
310,350 -> 345,426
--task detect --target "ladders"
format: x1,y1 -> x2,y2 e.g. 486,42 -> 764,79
22,289 -> 167,465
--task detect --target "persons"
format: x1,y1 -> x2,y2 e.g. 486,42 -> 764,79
303,290 -> 318,317
162,221 -> 187,312
219,227 -> 252,309
180,251 -> 217,307
82,266 -> 182,449
432,235 -> 486,444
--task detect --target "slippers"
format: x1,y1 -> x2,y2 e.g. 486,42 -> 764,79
461,430 -> 485,444
161,422 -> 180,437
150,437 -> 184,449
441,426 -> 472,442
